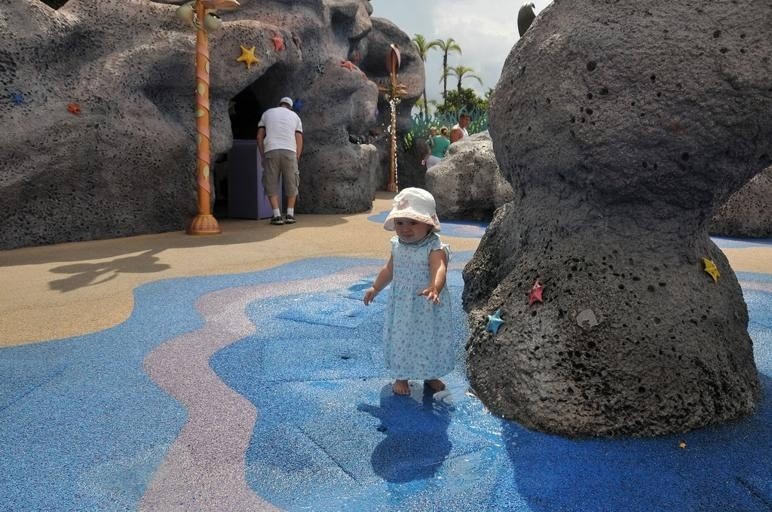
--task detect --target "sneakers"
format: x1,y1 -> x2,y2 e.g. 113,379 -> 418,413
285,215 -> 296,224
271,215 -> 284,224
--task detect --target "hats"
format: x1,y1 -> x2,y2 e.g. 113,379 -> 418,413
383,187 -> 441,232
280,97 -> 293,107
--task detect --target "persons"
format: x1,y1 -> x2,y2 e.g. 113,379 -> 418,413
421,126 -> 437,166
256,95 -> 305,225
449,110 -> 473,144
362,185 -> 458,397
425,125 -> 451,172
